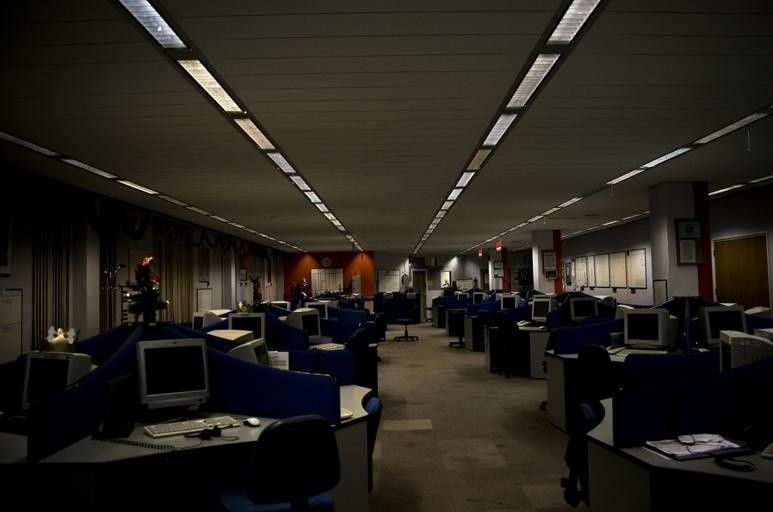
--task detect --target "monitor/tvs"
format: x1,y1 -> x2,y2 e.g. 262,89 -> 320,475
719,329 -> 773,374
138,338 -> 212,410
192,311 -> 223,332
229,312 -> 266,340
743,305 -> 773,328
501,295 -> 522,311
473,292 -> 489,305
698,305 -> 746,347
570,297 -> 598,322
22,350 -> 100,410
458,293 -> 470,301
280,308 -> 321,343
624,308 -> 678,349
207,329 -> 255,345
228,337 -> 272,367
531,298 -> 557,323
304,302 -> 328,320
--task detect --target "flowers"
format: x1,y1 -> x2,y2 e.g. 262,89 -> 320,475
129,254 -> 168,312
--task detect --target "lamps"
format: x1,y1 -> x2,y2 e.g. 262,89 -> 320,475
478,247 -> 483,257
496,239 -> 502,251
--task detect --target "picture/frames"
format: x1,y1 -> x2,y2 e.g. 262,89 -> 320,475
197,244 -> 210,283
240,269 -> 247,281
494,260 -> 503,269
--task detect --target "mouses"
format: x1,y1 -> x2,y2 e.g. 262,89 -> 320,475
539,325 -> 545,329
243,417 -> 261,426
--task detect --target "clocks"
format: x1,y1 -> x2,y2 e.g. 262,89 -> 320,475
321,257 -> 331,267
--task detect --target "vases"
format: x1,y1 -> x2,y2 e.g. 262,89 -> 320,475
142,311 -> 157,323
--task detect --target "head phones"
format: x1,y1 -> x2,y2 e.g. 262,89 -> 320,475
184,425 -> 222,439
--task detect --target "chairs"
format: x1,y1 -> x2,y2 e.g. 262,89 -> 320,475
392,298 -> 418,342
424,299 -> 434,322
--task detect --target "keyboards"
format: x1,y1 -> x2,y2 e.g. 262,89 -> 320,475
616,349 -> 669,357
144,415 -> 240,438
308,343 -> 346,352
